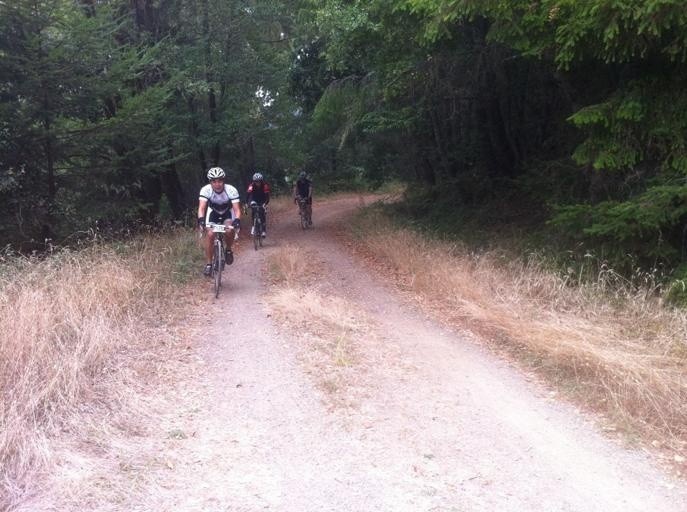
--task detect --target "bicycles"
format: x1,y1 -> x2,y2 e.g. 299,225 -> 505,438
294,195 -> 312,230
247,203 -> 268,250
200,221 -> 241,298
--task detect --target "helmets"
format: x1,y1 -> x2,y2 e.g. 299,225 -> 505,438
207,167 -> 225,181
252,173 -> 263,181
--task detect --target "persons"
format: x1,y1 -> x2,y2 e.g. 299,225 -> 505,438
197,167 -> 241,275
244,172 -> 270,237
294,172 -> 313,224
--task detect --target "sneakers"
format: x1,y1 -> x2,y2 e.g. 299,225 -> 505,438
225,248 -> 233,264
262,232 -> 266,238
205,264 -> 212,273
